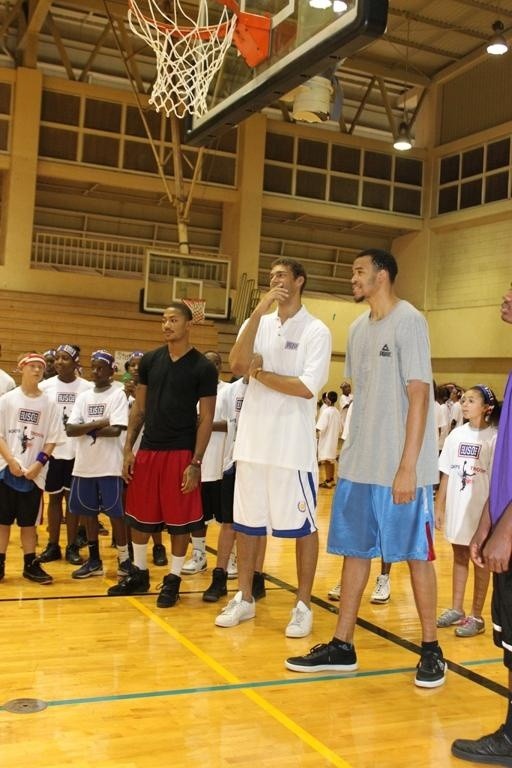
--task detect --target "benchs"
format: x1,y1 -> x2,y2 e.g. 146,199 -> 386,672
1,290 -> 240,388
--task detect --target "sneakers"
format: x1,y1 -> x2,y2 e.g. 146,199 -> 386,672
320,477 -> 336,489
0,521 -> 265,628
284,574 -> 485,689
452,724 -> 511,766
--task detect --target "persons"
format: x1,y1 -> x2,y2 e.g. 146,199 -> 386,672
0,302 -> 271,609
305,373 -> 500,638
450,287 -> 512,765
214,256 -> 332,638
286,248 -> 447,690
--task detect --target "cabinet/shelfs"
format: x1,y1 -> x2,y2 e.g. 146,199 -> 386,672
34,207 -> 362,284
